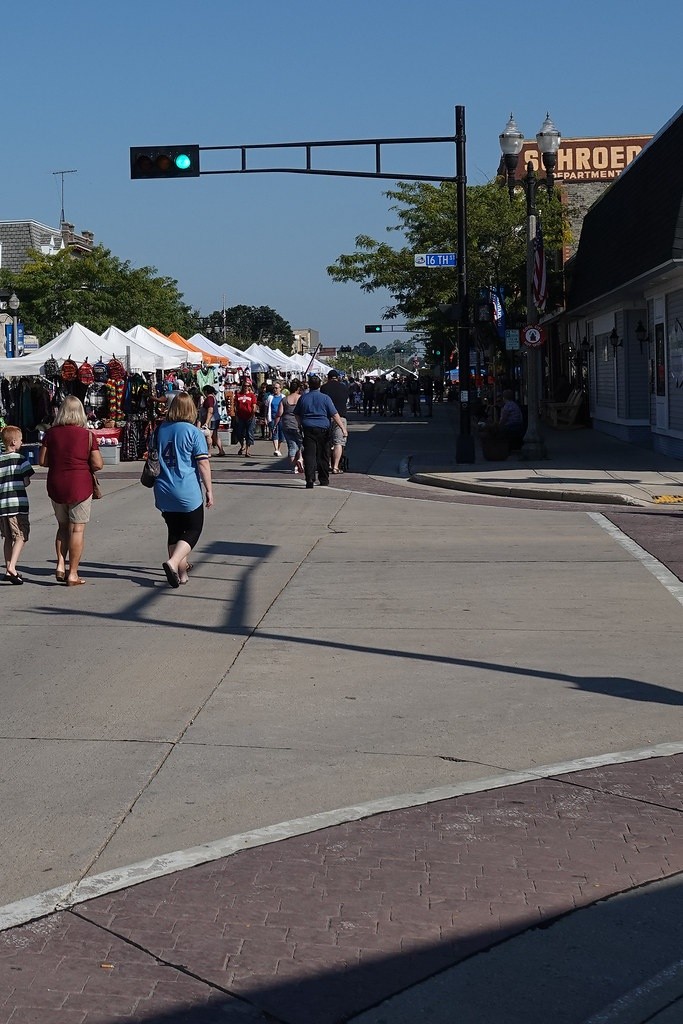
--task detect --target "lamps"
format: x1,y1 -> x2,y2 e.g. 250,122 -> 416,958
635,319 -> 659,356
581,341 -> 594,361
609,327 -> 623,357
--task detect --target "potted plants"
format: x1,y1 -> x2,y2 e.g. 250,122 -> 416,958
478,418 -> 526,461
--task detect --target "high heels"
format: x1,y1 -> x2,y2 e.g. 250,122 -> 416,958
56,571 -> 66,581
66,576 -> 85,585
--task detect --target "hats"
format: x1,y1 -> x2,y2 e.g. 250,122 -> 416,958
327,370 -> 339,379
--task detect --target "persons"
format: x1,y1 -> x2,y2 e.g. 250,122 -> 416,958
149,393 -> 213,587
479,389 -> 523,459
0,426 -> 35,585
149,370 -> 459,489
40,396 -> 103,586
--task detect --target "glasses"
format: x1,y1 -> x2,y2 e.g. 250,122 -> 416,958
242,385 -> 250,388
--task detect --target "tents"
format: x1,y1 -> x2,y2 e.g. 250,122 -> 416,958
0,321 -> 419,382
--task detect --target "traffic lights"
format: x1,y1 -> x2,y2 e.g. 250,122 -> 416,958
130,145 -> 199,178
395,348 -> 405,353
365,325 -> 382,333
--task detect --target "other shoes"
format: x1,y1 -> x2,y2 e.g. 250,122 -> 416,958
292,459 -> 304,474
321,482 -> 328,486
274,451 -> 282,457
215,451 -> 225,457
245,453 -> 251,457
306,482 -> 313,488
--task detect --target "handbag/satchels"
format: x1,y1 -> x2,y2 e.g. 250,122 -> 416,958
89,430 -> 102,499
231,429 -> 238,445
201,426 -> 210,437
141,421 -> 163,487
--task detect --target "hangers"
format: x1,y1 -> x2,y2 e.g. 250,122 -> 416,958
202,366 -> 207,371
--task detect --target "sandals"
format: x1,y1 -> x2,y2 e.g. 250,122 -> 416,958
332,468 -> 344,473
186,562 -> 193,572
3,572 -> 23,585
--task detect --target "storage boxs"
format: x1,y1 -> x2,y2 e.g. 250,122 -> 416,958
99,445 -> 121,465
218,432 -> 231,447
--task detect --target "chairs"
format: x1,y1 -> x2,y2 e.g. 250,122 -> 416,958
538,384 -> 586,430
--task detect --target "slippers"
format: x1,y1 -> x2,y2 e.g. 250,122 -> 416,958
162,562 -> 180,588
180,580 -> 189,584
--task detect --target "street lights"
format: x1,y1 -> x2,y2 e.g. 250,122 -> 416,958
499,111 -> 560,462
205,322 -> 221,347
8,292 -> 21,356
